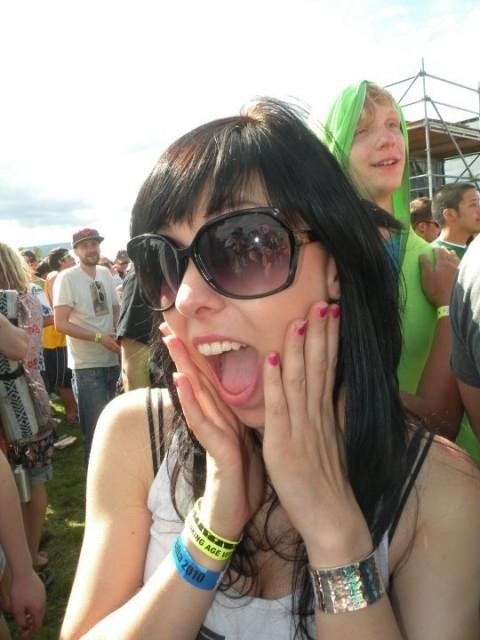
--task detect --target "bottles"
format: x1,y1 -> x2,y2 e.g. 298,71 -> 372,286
12,456 -> 31,504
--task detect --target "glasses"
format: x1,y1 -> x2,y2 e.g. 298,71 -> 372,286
127,207 -> 322,312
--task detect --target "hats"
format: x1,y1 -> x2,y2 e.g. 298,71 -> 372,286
72,229 -> 104,247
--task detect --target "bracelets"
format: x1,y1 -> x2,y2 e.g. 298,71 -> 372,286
184,496 -> 242,560
308,550 -> 387,614
171,536 -> 226,591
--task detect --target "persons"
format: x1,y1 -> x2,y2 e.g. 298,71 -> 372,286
62,93 -> 480,638
223,226 -> 290,282
1,228 -> 162,639
323,81 -> 480,460
